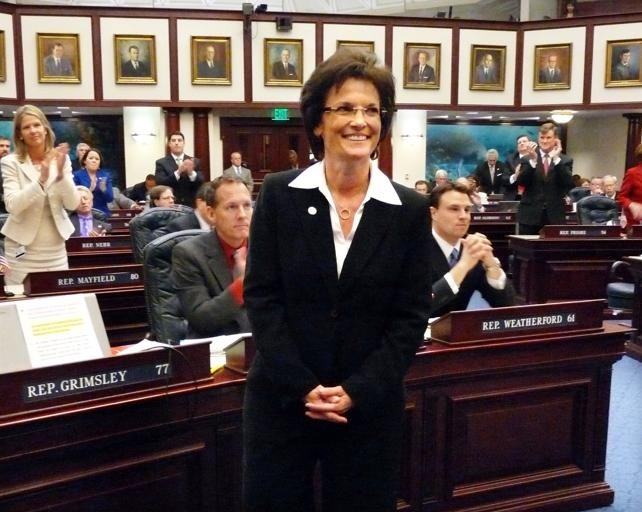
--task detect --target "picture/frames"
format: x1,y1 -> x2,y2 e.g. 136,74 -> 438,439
403,42 -> 441,89
469,44 -> 507,92
336,41 -> 374,54
532,43 -> 572,92
191,36 -> 233,85
263,38 -> 304,87
115,34 -> 159,85
604,37 -> 640,88
1,30 -> 8,82
36,32 -> 82,84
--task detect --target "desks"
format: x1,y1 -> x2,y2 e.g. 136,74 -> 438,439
462,190 -> 639,303
22,206 -> 156,345
1,297 -> 638,510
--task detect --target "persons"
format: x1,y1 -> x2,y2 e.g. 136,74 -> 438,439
230,46 -> 434,512
174,174 -> 255,334
121,46 -> 148,77
43,41 -> 76,76
271,48 -> 297,80
1,105 -> 85,294
613,48 -> 634,80
618,143 -> 642,233
408,51 -> 436,83
475,53 -> 500,84
512,122 -> 576,235
422,178 -> 517,315
197,44 -> 225,77
539,54 -> 562,82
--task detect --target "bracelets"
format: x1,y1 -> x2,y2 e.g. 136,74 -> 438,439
481,256 -> 501,272
37,178 -> 45,189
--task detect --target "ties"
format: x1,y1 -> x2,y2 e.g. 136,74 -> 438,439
448,248 -> 461,269
543,154 -> 550,178
237,167 -> 240,175
232,246 -> 247,282
80,216 -> 92,238
176,157 -> 183,166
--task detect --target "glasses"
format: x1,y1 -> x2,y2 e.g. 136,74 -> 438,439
322,105 -> 389,120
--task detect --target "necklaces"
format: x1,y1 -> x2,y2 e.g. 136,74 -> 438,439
325,183 -> 366,222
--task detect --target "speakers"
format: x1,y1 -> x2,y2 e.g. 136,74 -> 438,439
243,2 -> 253,15
